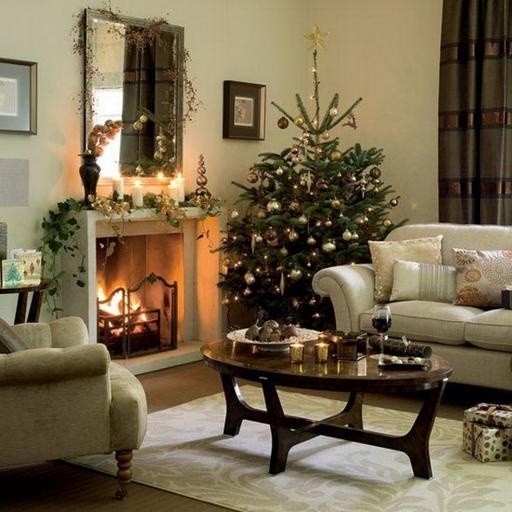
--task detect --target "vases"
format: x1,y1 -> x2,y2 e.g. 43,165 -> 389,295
78,153 -> 102,211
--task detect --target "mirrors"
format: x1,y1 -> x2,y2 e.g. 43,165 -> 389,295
83,8 -> 185,178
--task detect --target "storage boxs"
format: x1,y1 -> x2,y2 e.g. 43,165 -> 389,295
463,403 -> 512,463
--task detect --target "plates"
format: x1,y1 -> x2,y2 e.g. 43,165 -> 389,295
227,327 -> 321,351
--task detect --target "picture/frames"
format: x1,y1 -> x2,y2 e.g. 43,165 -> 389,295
222,80 -> 267,140
0,58 -> 38,135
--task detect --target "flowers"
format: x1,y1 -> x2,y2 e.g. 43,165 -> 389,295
87,115 -> 148,155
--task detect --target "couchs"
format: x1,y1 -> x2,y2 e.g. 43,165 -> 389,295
312,224 -> 512,390
0,315 -> 148,495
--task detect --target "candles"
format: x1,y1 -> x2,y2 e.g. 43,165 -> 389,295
175,177 -> 184,202
113,177 -> 123,200
132,186 -> 143,208
168,185 -> 179,207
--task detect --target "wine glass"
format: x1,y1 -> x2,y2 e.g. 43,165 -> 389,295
369,305 -> 392,358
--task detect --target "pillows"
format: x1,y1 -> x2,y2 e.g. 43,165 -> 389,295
368,234 -> 444,304
0,317 -> 30,353
452,248 -> 512,306
389,258 -> 458,303
